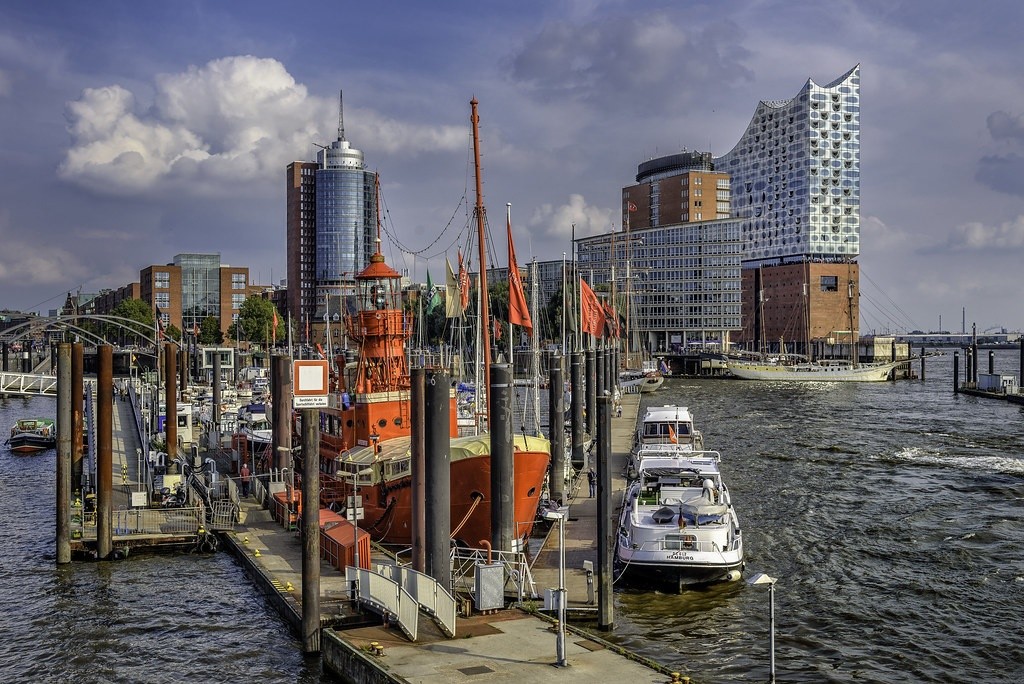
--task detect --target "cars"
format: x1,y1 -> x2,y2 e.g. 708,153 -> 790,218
252,377 -> 270,395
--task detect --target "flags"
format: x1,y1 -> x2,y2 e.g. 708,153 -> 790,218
580,279 -> 606,337
446,257 -> 462,318
427,267 -> 441,314
273,311 -> 278,328
237,319 -> 246,334
602,300 -> 628,339
629,202 -> 637,211
494,319 -> 502,339
182,319 -> 188,343
561,263 -> 575,336
194,323 -> 199,337
669,426 -> 677,443
507,221 -> 533,337
156,305 -> 164,339
457,247 -> 471,310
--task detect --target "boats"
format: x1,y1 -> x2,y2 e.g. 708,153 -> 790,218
264,91 -> 551,553
4,417 -> 57,455
725,254 -> 947,381
617,405 -> 745,592
619,260 -> 664,393
237,400 -> 272,443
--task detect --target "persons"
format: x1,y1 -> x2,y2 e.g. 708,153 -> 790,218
587,468 -> 596,498
118,377 -> 126,402
240,463 -> 249,499
112,379 -> 119,405
614,403 -> 623,417
